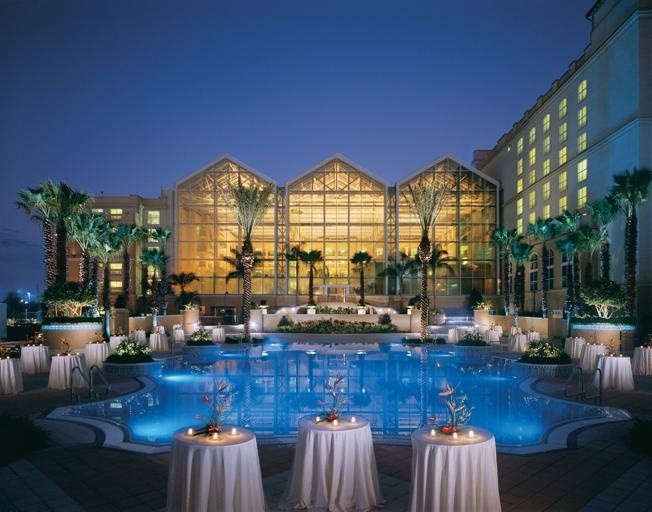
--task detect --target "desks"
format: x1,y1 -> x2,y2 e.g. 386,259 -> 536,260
486,325 -> 540,353
447,327 -> 458,343
283,413 -> 387,511
408,425 -> 502,511
165,422 -> 266,512
564,337 -> 652,392
131,323 -> 185,353
212,328 -> 225,343
0,334 -> 128,396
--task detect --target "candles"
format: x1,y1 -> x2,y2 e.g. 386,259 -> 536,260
316,416 -> 320,421
213,432 -> 218,440
452,432 -> 457,438
351,415 -> 355,423
469,429 -> 474,438
430,428 -> 436,436
188,427 -> 192,435
231,427 -> 236,435
333,419 -> 337,425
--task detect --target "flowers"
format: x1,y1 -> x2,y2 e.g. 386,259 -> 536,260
195,381 -> 231,434
314,372 -> 349,417
426,383 -> 475,435
